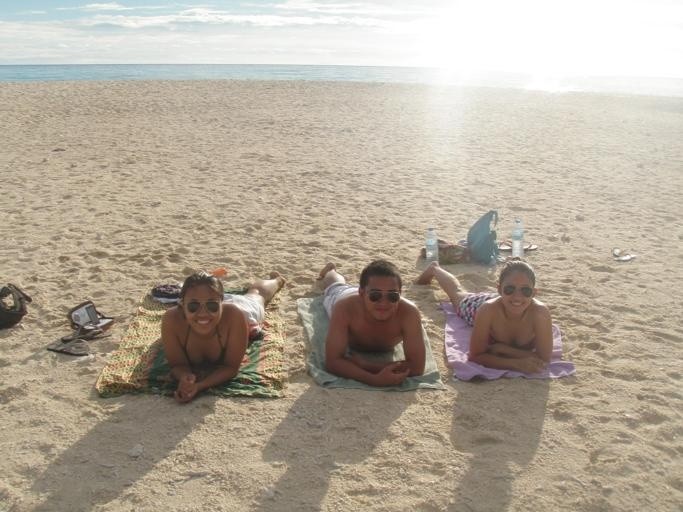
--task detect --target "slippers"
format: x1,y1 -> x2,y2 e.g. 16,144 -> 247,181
48,324 -> 111,355
497,239 -> 538,251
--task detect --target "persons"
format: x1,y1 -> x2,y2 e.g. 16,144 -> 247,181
316,260 -> 425,387
161,271 -> 286,402
413,260 -> 553,375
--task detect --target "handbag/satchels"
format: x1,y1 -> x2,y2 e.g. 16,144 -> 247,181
468,211 -> 498,262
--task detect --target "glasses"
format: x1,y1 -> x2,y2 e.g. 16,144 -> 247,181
368,290 -> 399,303
503,286 -> 532,297
188,302 -> 219,312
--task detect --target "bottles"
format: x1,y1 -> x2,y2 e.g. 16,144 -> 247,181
424,228 -> 439,265
511,218 -> 525,258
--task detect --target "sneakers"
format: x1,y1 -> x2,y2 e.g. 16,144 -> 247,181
152,285 -> 181,303
